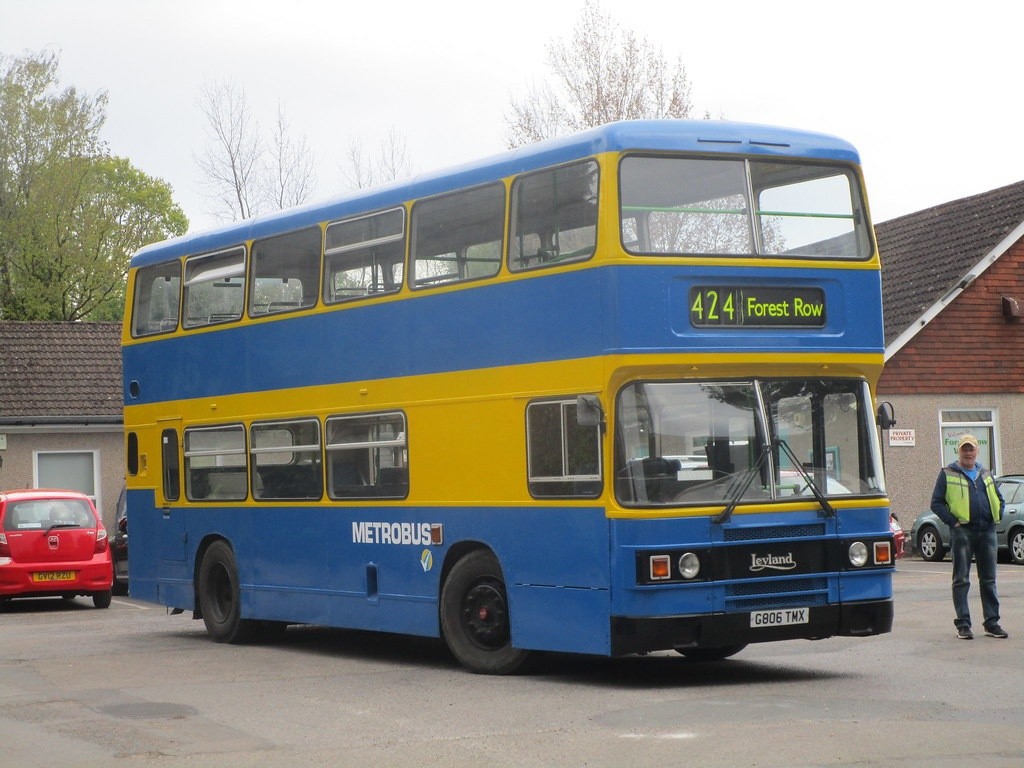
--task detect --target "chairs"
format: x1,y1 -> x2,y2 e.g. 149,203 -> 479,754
49,506 -> 72,522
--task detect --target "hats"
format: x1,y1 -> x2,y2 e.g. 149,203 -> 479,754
957,434 -> 978,449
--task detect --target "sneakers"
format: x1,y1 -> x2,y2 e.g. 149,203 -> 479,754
984,625 -> 1008,638
956,626 -> 974,639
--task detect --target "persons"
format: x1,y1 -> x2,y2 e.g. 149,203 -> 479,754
930,435 -> 1009,640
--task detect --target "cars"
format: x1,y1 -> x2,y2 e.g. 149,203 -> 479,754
777,471 -> 905,558
107,483 -> 130,586
0,490 -> 115,609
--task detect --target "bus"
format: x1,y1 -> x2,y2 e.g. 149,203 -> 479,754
119,120 -> 899,678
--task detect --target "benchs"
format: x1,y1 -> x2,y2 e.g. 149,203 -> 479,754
159,280 -> 402,333
172,465 -> 406,501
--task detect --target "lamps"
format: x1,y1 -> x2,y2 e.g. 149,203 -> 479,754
940,274 -> 976,302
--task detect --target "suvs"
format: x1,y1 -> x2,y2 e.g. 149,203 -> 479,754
909,474 -> 1024,565
